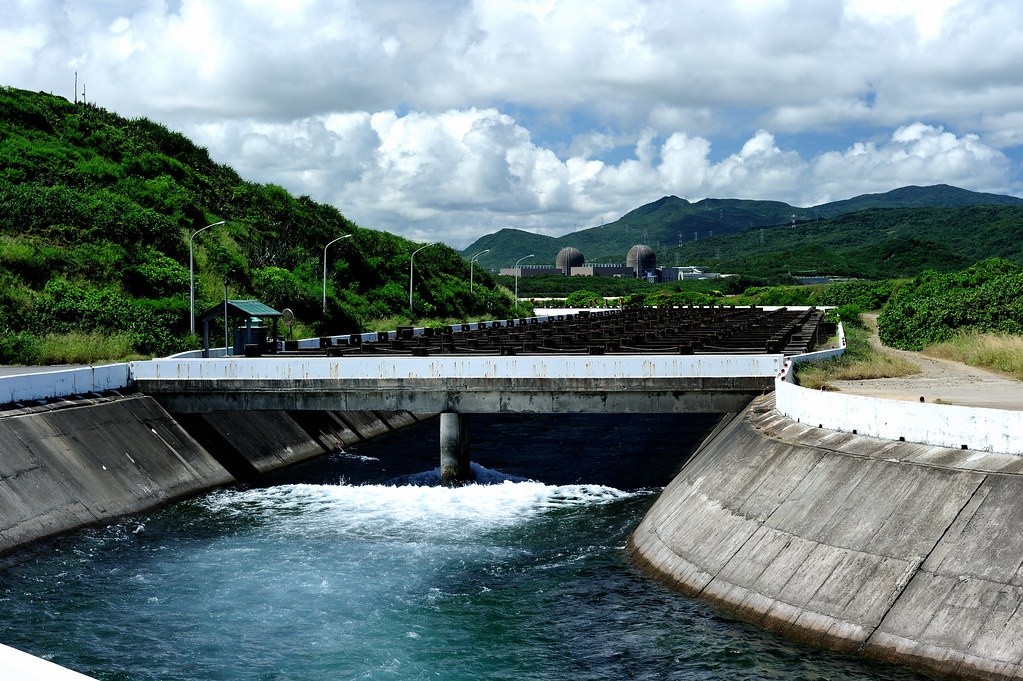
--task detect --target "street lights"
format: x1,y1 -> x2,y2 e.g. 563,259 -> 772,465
410,243 -> 437,313
515,255 -> 536,308
189,220 -> 226,337
322,234 -> 352,317
470,249 -> 491,293
